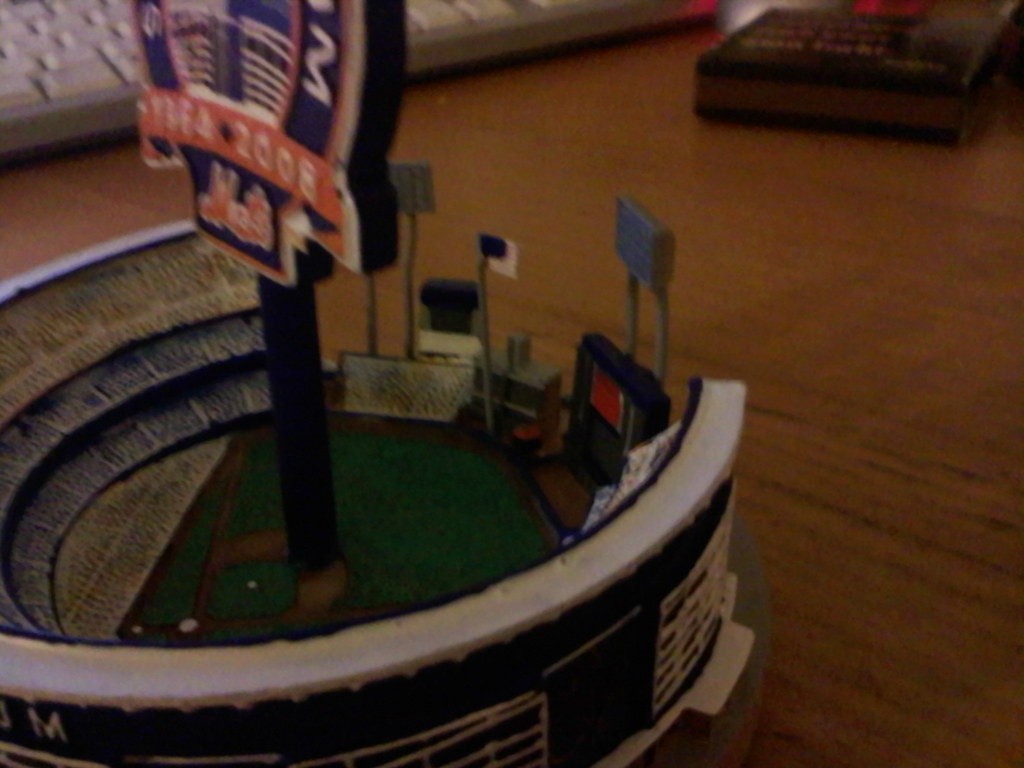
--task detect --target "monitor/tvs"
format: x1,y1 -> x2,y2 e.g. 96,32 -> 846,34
591,363 -> 625,434
